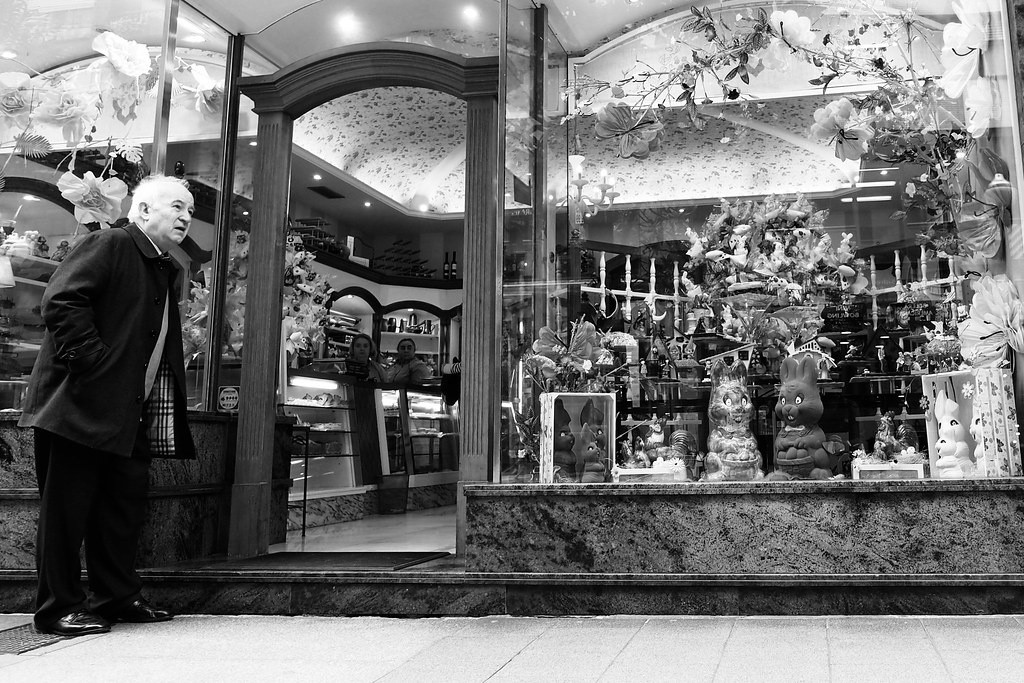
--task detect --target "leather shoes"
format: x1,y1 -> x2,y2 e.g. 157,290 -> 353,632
93,598 -> 175,622
33,612 -> 111,636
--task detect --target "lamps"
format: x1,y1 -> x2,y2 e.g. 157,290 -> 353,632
0,256 -> 16,288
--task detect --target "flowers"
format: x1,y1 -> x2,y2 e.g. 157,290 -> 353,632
531,0 -> 1024,372
0,29 -> 224,229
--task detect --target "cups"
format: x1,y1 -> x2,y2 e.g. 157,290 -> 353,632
1,220 -> 17,238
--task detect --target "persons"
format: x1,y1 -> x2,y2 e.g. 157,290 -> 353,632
21,176 -> 198,635
387,338 -> 431,384
347,333 -> 387,383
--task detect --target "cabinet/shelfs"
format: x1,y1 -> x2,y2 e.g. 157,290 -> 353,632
327,326 -> 360,348
220,363 -> 366,502
355,381 -> 460,490
0,255 -> 62,352
380,331 -> 439,356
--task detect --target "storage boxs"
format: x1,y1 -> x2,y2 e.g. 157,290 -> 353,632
921,369 -> 1023,478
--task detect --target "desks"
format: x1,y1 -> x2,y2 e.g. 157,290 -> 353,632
856,415 -> 925,452
659,377 -> 846,473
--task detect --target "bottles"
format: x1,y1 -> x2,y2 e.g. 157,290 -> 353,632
443,251 -> 450,279
450,251 -> 458,279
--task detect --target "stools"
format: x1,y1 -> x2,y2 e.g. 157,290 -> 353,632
539,392 -> 616,484
